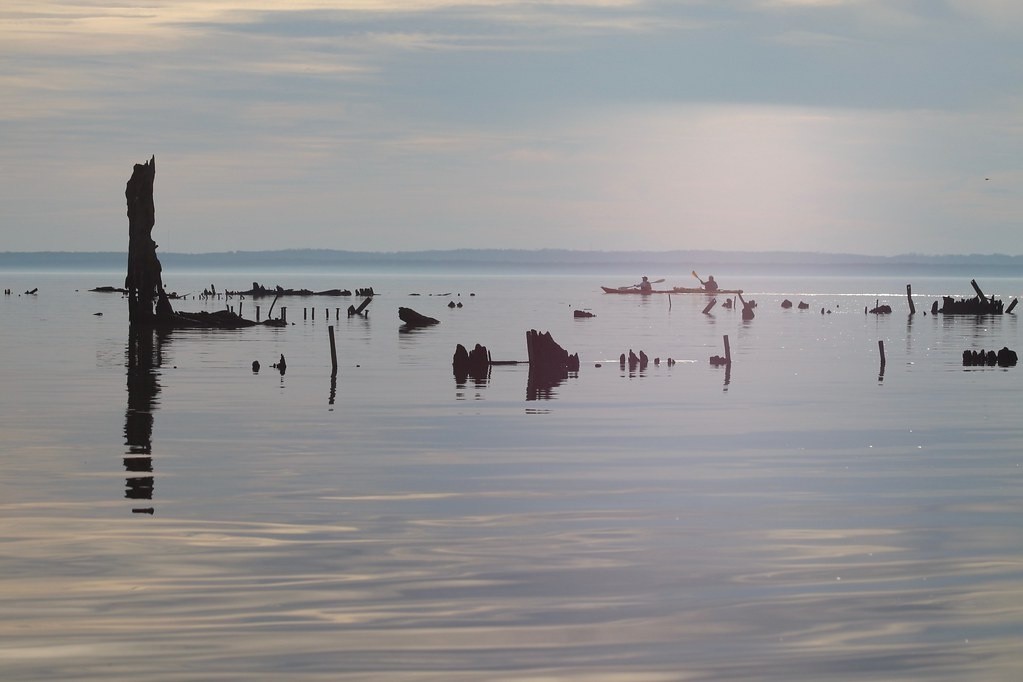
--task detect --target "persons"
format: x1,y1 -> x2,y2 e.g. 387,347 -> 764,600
636,276 -> 651,295
701,276 -> 718,291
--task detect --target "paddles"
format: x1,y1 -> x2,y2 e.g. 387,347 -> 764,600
618,278 -> 666,289
692,270 -> 703,283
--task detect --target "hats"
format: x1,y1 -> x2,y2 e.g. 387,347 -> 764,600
642,275 -> 647,278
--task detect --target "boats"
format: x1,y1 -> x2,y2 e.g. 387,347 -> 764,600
672,286 -> 742,294
600,286 -> 677,294
